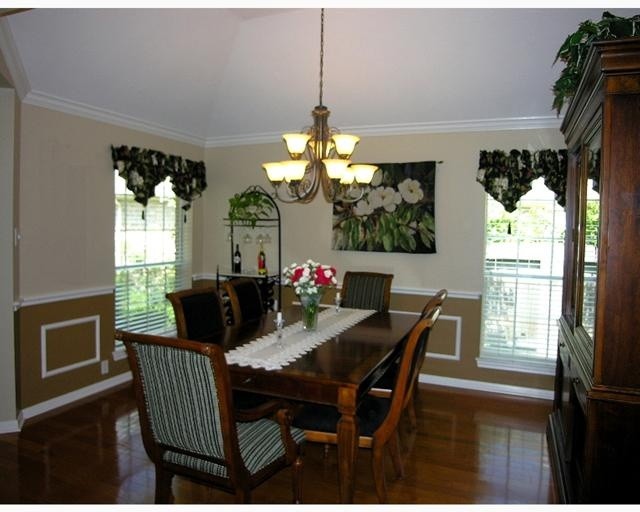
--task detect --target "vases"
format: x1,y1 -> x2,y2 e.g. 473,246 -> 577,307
300,288 -> 328,331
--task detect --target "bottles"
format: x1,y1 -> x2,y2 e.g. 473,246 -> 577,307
257,240 -> 268,274
233,243 -> 241,274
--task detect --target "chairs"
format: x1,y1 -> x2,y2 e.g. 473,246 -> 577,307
166,286 -> 293,417
114,330 -> 307,504
340,271 -> 394,310
291,307 -> 441,504
224,278 -> 263,324
367,289 -> 448,428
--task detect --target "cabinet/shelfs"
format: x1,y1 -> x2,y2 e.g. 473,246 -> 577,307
546,37 -> 640,505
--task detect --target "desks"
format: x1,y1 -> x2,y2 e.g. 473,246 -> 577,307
215,273 -> 281,325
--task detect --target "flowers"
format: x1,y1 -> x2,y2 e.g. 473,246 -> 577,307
229,191 -> 274,228
283,259 -> 338,329
333,169 -> 435,252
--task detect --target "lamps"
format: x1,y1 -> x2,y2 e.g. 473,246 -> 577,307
262,8 -> 378,205
